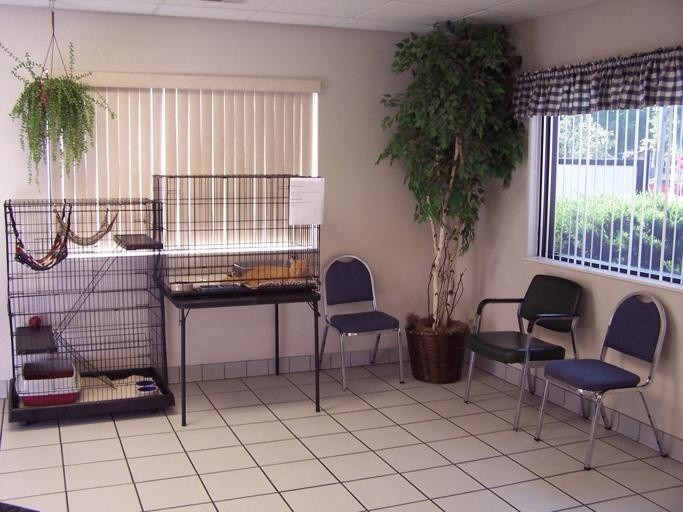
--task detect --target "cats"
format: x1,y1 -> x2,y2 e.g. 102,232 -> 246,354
220,256 -> 312,289
226,264 -> 253,277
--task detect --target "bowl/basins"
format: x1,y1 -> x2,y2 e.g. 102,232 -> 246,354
170,281 -> 194,293
135,386 -> 159,397
135,379 -> 156,388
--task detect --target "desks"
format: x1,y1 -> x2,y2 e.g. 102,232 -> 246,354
151,274 -> 322,426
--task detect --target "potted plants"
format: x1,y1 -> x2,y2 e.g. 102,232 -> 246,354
0,40 -> 118,195
373,15 -> 527,384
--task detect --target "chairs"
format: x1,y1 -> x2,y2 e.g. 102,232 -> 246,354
464,274 -> 585,432
534,292 -> 668,470
320,254 -> 405,391
